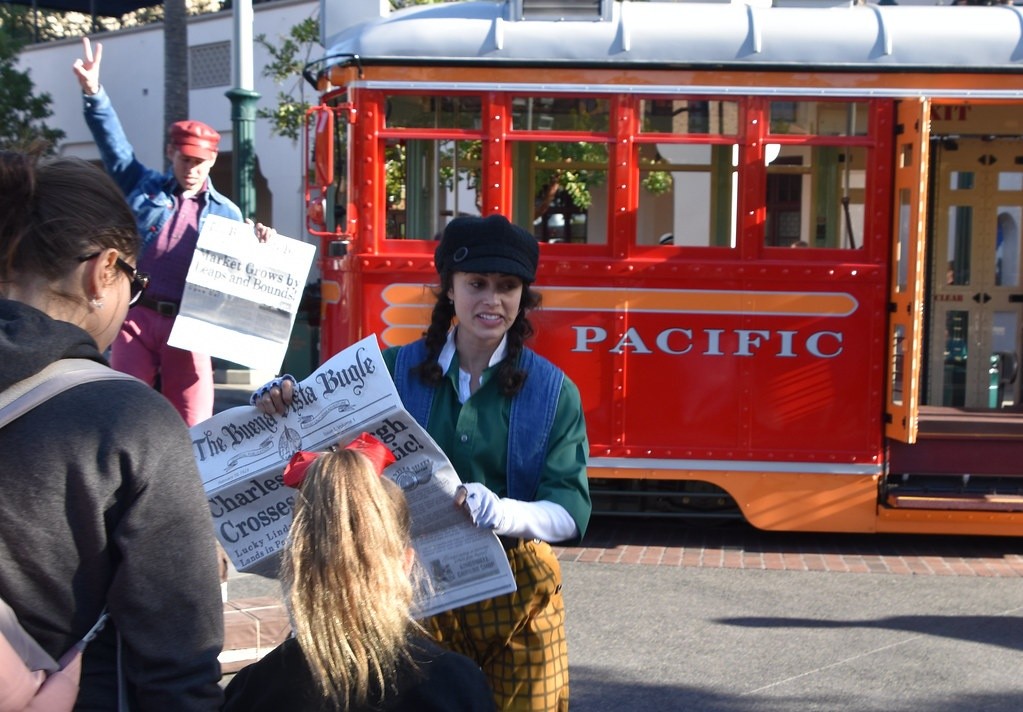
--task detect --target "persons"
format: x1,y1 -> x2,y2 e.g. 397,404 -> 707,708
246,206 -> 593,712
1,144 -> 228,712
211,448 -> 500,712
71,33 -> 275,428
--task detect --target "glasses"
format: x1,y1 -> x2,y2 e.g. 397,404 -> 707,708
79,250 -> 149,307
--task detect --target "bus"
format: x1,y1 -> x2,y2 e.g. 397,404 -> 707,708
297,0 -> 1023,542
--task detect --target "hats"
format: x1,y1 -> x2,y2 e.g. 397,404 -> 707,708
434,214 -> 539,285
168,120 -> 220,160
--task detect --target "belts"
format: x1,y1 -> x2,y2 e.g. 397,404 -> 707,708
138,295 -> 180,317
